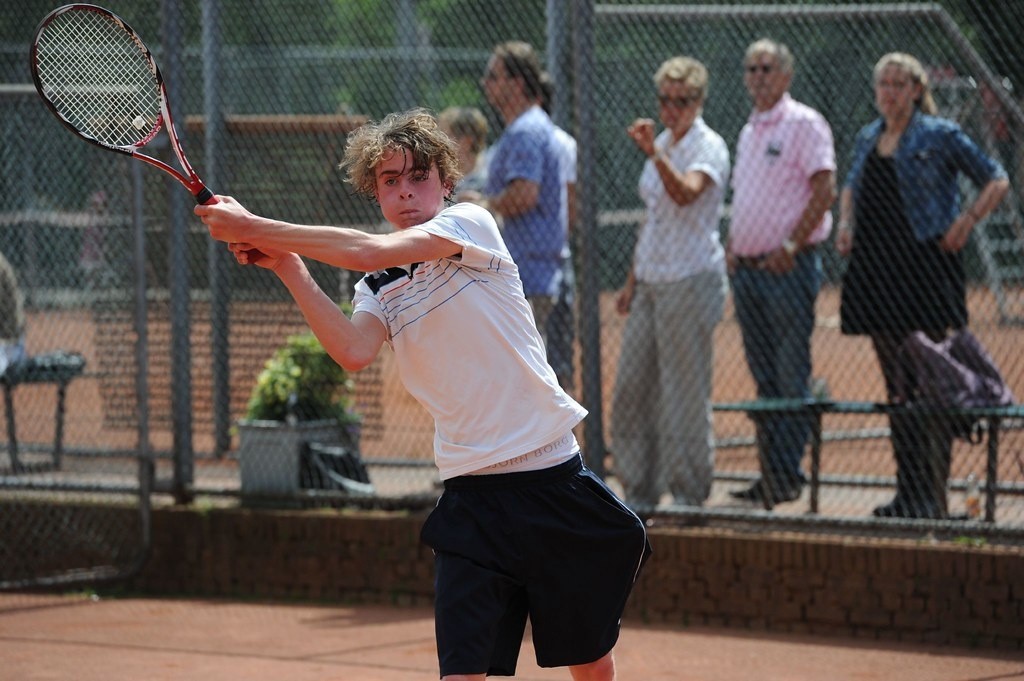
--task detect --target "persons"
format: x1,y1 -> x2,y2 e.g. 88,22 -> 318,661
80,190 -> 110,291
819,51 -> 1008,520
606,57 -> 731,520
478,40 -> 573,359
721,37 -> 837,502
193,104 -> 645,681
436,108 -> 498,214
1,251 -> 25,378
536,70 -> 580,398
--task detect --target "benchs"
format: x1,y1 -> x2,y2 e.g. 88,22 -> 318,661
708,400 -> 1023,522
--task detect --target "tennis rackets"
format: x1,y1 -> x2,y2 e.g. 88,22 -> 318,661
29,2 -> 261,264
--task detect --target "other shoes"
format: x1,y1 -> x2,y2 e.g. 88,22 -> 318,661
726,479 -> 807,506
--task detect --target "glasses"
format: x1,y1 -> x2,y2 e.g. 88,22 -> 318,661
659,95 -> 695,110
744,66 -> 785,74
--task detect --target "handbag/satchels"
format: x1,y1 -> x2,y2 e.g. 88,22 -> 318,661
898,326 -> 1016,414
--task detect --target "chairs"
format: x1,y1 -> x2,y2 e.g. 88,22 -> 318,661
0,254 -> 84,474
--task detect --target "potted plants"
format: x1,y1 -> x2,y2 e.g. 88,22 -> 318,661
235,334 -> 359,494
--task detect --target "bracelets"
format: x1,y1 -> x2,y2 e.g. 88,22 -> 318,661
966,205 -> 981,226
782,237 -> 799,257
650,147 -> 663,161
